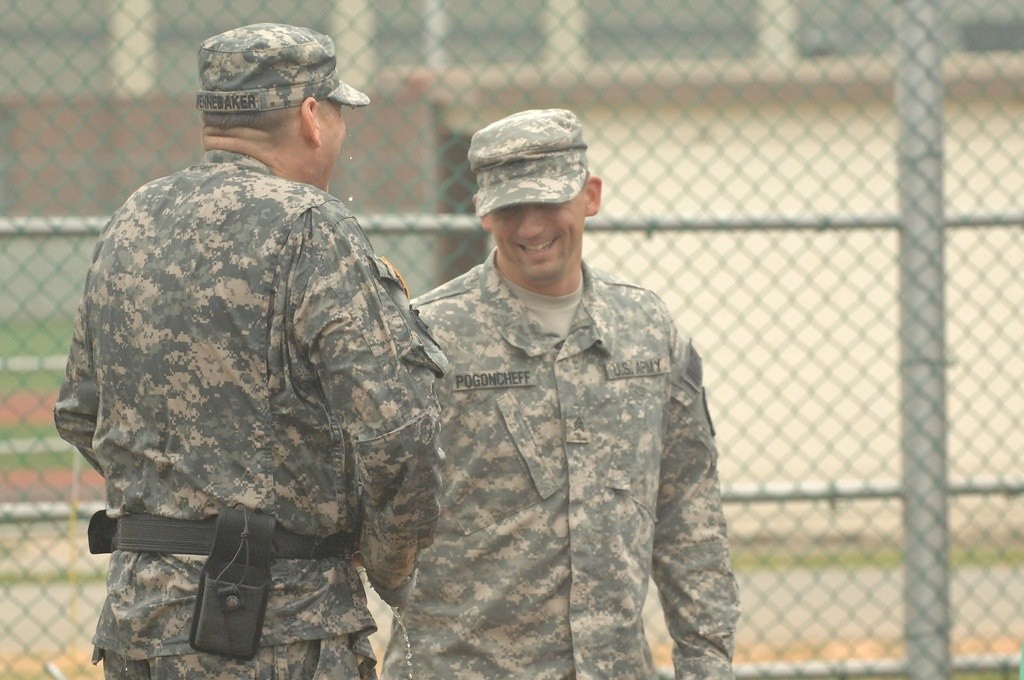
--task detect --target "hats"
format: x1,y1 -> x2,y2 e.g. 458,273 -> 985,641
466,107 -> 590,219
193,22 -> 370,113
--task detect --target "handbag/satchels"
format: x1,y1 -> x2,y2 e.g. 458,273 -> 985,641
189,508 -> 277,662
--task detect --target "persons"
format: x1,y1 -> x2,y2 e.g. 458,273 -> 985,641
377,107 -> 745,680
52,21 -> 453,679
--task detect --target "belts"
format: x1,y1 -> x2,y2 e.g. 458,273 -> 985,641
86,508 -> 354,556
